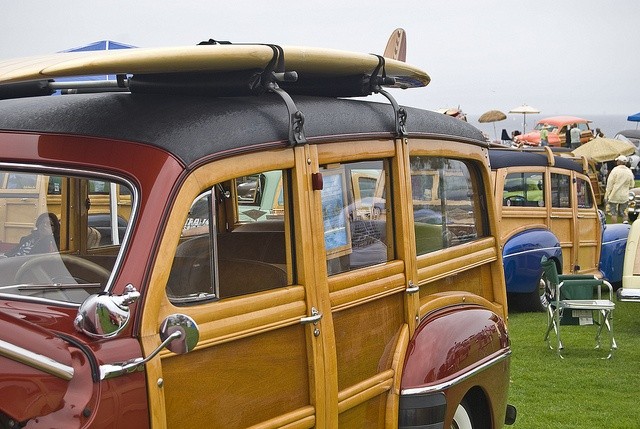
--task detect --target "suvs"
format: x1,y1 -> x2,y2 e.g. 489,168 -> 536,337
514,118 -> 593,149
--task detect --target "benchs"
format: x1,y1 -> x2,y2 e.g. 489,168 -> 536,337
231,221 -> 461,257
167,231 -> 387,268
65,255 -> 286,301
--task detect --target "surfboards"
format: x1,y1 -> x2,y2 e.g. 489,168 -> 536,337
0,42 -> 430,99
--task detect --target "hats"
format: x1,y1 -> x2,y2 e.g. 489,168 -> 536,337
615,155 -> 628,162
542,123 -> 551,128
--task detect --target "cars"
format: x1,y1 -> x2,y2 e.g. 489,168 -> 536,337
0,93 -> 517,429
491,166 -> 631,308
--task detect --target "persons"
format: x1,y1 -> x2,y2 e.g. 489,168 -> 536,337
541,122 -> 551,144
605,156 -> 634,224
569,123 -> 581,149
2,212 -> 60,258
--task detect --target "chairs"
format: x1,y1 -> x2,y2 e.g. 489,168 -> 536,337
539,260 -> 617,360
88,226 -> 102,248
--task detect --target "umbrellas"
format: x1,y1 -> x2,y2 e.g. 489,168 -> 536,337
479,111 -> 507,143
508,105 -> 540,134
569,135 -> 636,185
